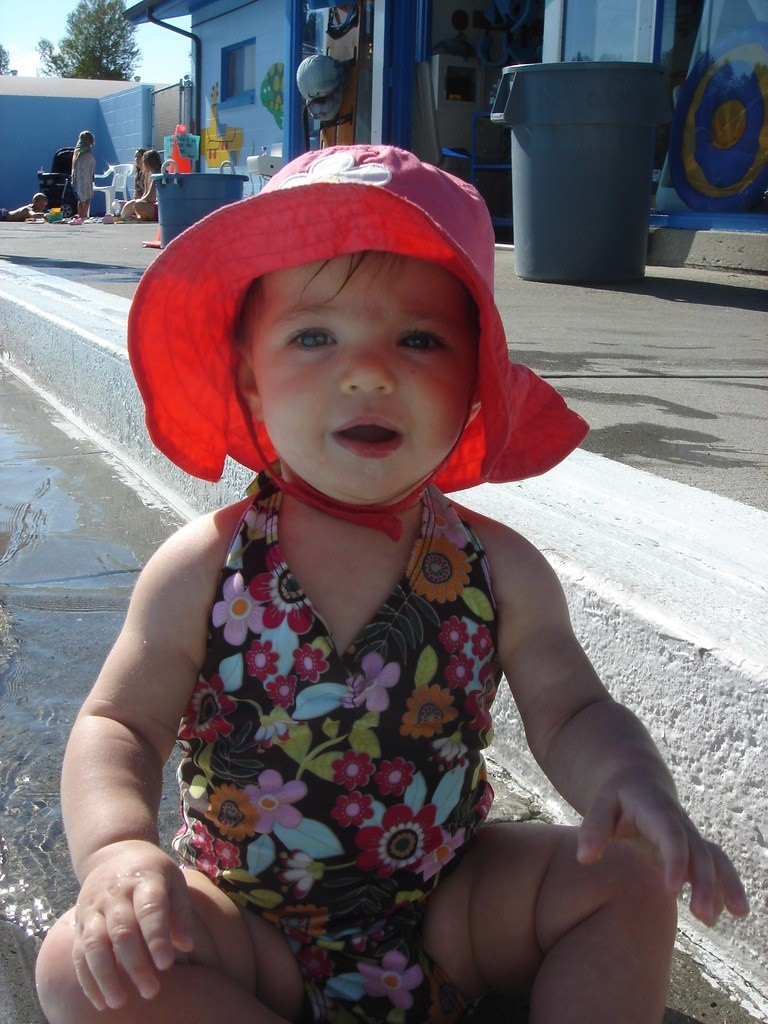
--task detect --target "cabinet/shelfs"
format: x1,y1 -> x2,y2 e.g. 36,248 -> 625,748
471,112 -> 512,226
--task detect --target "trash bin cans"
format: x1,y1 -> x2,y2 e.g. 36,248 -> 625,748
488,62 -> 662,287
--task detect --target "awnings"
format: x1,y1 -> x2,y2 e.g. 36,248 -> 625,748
122,0 -> 219,38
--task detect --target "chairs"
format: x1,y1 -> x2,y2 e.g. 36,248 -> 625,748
86,163 -> 133,218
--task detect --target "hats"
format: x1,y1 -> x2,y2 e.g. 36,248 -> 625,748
124,144 -> 590,546
295,51 -> 348,153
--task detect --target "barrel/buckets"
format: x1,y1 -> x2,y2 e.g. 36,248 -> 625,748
149,160 -> 249,249
490,60 -> 678,284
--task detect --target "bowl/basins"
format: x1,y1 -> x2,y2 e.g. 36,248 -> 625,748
51,208 -> 61,212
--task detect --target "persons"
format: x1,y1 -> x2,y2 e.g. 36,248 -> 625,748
0,193 -> 47,221
71,131 -> 96,220
36,146 -> 749,1024
116,148 -> 168,221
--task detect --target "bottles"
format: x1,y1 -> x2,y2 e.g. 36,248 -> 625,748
111,199 -> 121,214
